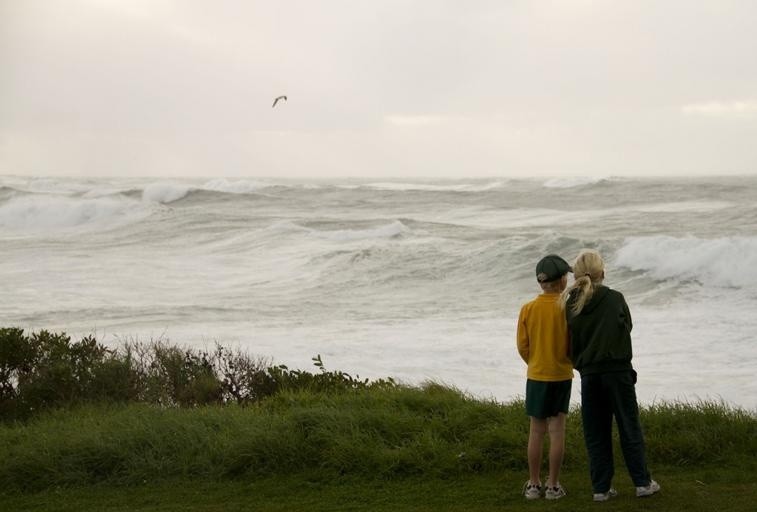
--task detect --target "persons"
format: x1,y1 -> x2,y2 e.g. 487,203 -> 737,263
561,247 -> 660,503
512,253 -> 576,502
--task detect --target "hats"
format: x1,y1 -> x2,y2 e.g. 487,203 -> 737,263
535,255 -> 574,283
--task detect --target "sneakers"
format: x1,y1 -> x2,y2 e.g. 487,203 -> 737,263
544,479 -> 566,500
636,480 -> 660,497
593,488 -> 617,502
525,480 -> 542,500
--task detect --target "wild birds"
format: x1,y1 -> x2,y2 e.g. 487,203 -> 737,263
273,95 -> 287,108
